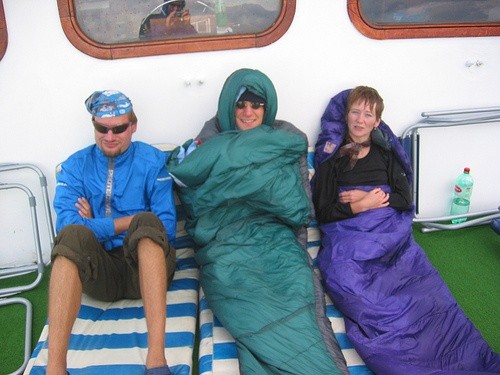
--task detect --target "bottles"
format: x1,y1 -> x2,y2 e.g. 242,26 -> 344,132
216,0 -> 228,31
451,167 -> 473,223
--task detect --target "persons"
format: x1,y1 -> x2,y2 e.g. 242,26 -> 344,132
138,0 -> 195,38
45,90 -> 179,375
311,86 -> 415,222
234,87 -> 266,131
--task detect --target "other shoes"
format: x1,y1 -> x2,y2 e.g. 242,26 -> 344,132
145,363 -> 172,375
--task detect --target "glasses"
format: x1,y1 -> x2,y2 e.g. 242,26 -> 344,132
237,101 -> 264,109
92,121 -> 129,134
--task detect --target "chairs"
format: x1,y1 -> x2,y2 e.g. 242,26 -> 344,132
201,146 -> 374,375
23,144 -> 199,375
